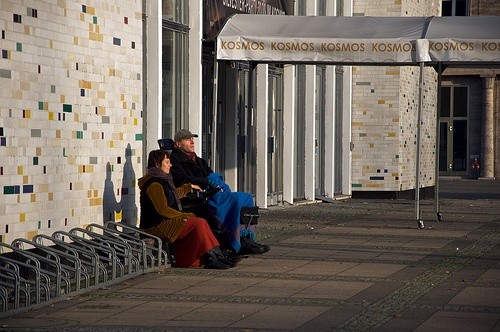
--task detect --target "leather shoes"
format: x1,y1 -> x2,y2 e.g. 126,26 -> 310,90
240,237 -> 271,254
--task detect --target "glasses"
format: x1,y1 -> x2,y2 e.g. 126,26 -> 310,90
182,137 -> 195,142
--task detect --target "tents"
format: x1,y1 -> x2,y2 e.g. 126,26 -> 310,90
211,14 -> 500,229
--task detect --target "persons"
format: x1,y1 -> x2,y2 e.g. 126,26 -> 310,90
170,129 -> 270,261
138,150 -> 237,269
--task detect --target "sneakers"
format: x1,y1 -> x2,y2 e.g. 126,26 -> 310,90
203,253 -> 238,268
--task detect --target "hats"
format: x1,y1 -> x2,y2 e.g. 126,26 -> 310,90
174,129 -> 198,143
